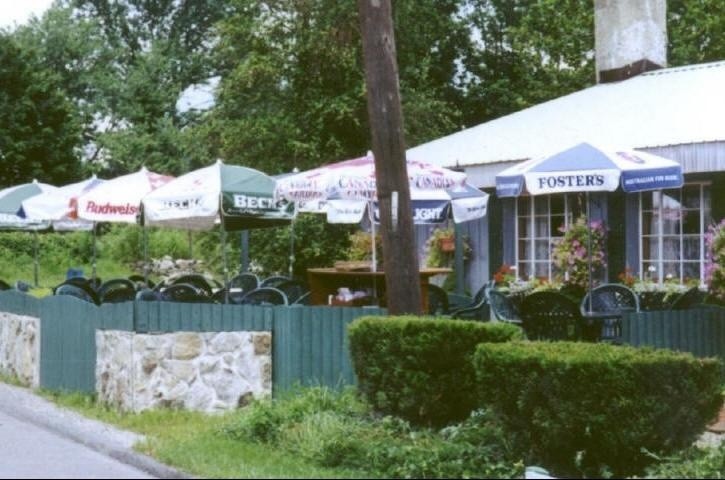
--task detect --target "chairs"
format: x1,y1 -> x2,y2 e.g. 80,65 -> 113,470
52,269 -> 310,307
446,279 -> 497,321
578,284 -> 641,340
489,290 -> 522,327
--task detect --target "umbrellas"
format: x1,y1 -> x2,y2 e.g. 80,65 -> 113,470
653,191 -> 687,222
277,151 -> 467,307
298,182 -> 488,229
494,137 -> 686,316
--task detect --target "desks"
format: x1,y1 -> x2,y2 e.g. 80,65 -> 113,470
526,310 -> 622,343
306,263 -> 452,314
